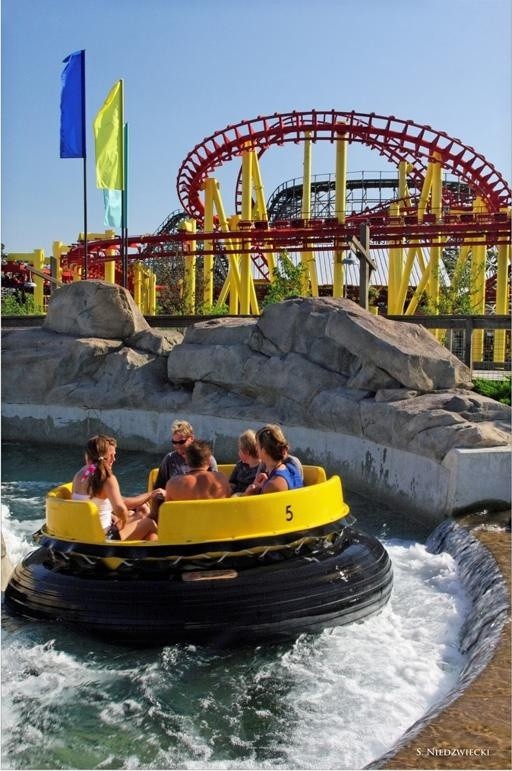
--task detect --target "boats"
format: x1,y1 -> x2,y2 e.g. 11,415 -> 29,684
1,461 -> 397,649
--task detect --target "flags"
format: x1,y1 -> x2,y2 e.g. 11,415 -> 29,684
100,122 -> 128,229
92,80 -> 124,191
60,49 -> 86,160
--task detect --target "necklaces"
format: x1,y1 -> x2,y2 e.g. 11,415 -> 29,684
188,469 -> 205,474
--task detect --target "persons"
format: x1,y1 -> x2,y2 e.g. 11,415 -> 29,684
162,439 -> 231,502
255,423 -> 305,482
152,420 -> 218,505
240,428 -> 304,496
71,437 -> 158,541
95,433 -> 166,524
229,427 -> 262,496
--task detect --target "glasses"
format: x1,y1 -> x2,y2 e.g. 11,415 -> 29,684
171,439 -> 186,444
